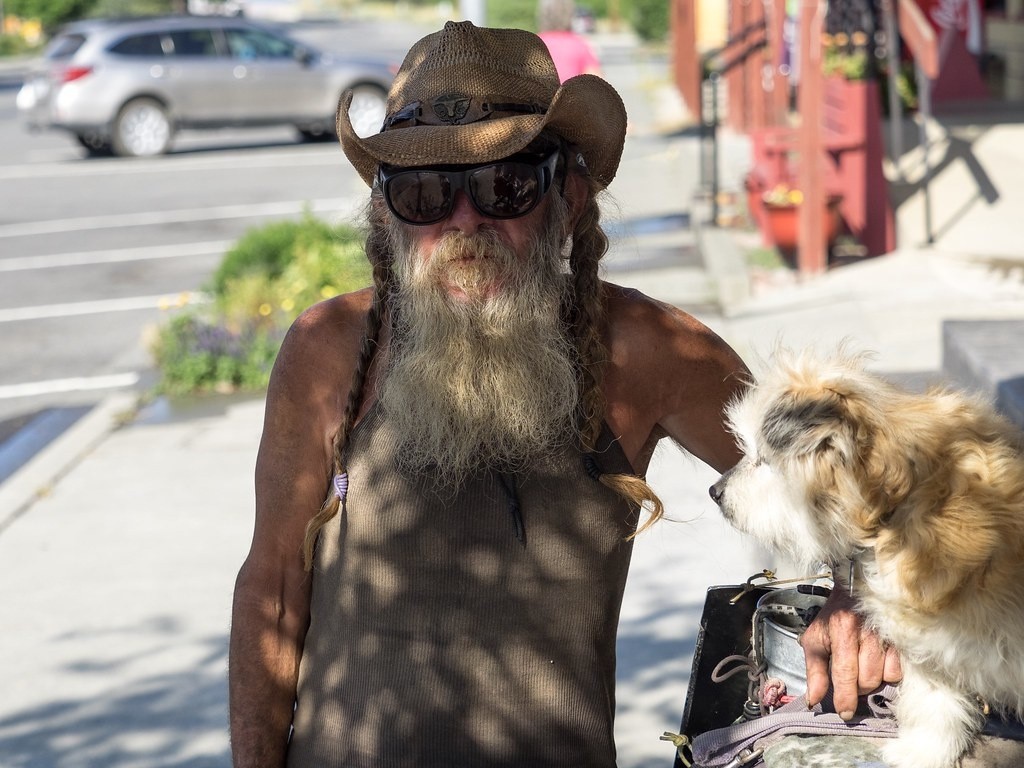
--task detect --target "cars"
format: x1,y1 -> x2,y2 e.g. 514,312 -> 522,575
14,12 -> 401,158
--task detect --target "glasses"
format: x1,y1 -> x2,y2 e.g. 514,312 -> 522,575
376,139 -> 561,228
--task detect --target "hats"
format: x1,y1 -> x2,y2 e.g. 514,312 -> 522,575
336,20 -> 627,193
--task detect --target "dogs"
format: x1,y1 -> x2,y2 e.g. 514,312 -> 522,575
706,332 -> 1024,768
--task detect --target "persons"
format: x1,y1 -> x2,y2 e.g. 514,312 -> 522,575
230,21 -> 902,768
